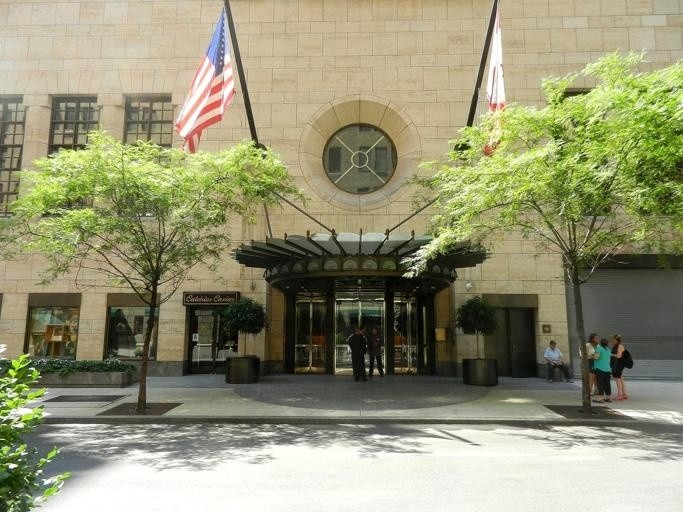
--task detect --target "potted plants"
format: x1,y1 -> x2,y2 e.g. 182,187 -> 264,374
218,296 -> 270,385
452,294 -> 502,387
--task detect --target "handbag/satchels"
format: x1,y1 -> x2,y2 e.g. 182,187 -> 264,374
620,349 -> 634,369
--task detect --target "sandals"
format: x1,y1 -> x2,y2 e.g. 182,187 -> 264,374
589,391 -> 628,403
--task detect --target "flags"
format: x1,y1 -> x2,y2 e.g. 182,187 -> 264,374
172,3 -> 241,156
482,11 -> 508,161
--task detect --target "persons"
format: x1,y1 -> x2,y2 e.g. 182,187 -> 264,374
346,324 -> 366,343
579,333 -> 600,397
544,339 -> 574,383
593,338 -> 612,403
610,334 -> 628,400
350,327 -> 368,381
366,327 -> 385,377
